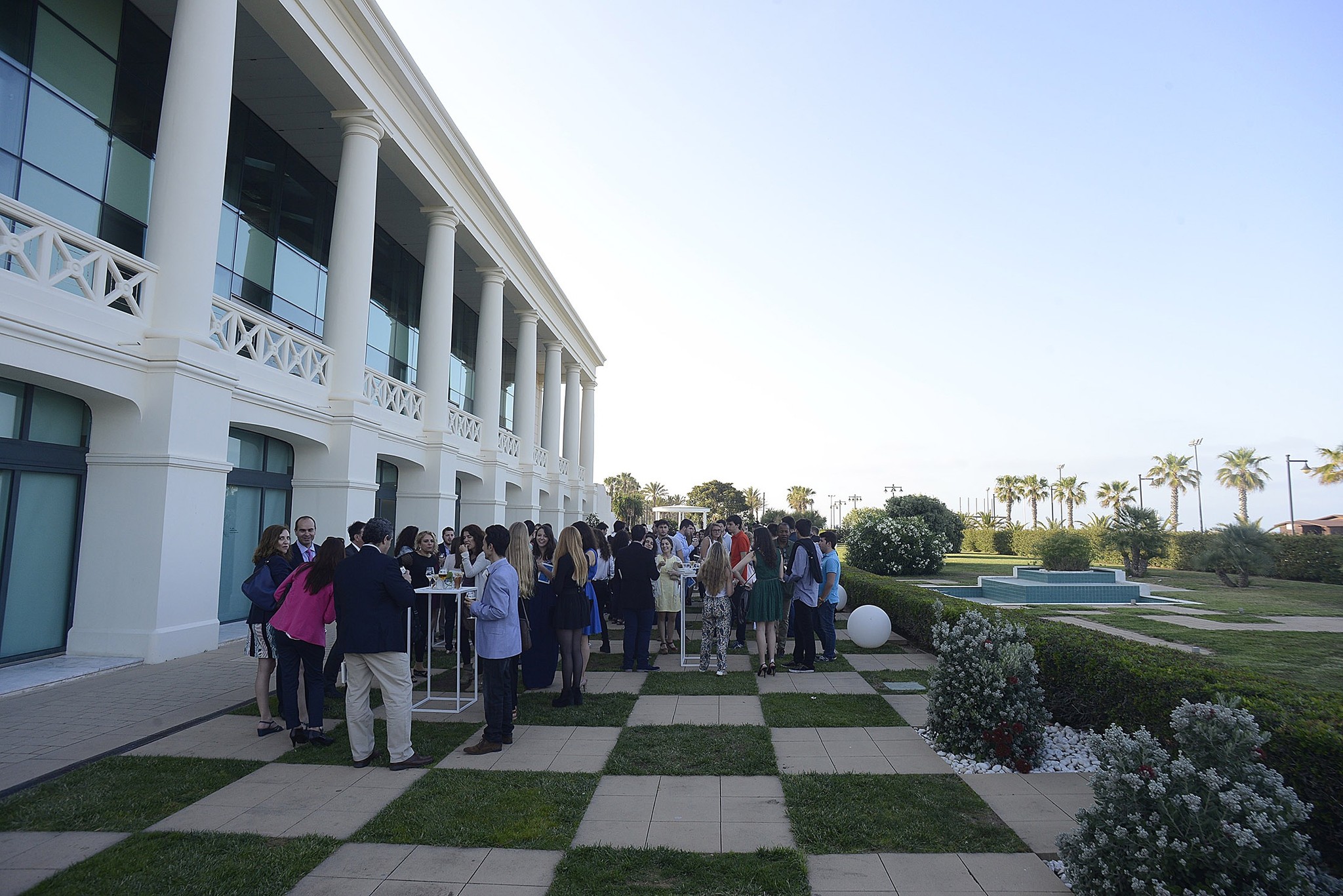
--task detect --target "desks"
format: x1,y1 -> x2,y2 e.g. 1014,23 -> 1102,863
406,585 -> 479,713
680,573 -> 718,668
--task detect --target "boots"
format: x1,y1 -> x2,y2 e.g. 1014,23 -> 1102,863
600,639 -> 610,653
460,663 -> 483,692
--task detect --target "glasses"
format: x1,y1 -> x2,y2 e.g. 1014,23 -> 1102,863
711,529 -> 722,533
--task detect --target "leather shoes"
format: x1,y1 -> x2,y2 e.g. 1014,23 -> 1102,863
501,732 -> 513,744
325,682 -> 348,698
389,752 -> 435,770
353,749 -> 380,768
463,738 -> 502,755
636,658 -> 661,672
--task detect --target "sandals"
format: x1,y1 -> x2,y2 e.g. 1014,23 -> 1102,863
612,618 -> 625,625
411,675 -> 418,683
413,668 -> 433,678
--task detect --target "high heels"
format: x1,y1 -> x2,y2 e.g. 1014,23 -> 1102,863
552,689 -> 570,707
257,721 -> 284,737
570,675 -> 588,692
290,727 -> 309,747
569,687 -> 582,705
305,730 -> 336,747
666,640 -> 678,653
757,662 -> 768,678
766,662 -> 776,677
660,642 -> 668,655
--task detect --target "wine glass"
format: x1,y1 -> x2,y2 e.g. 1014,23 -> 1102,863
689,561 -> 695,569
465,591 -> 477,619
426,566 -> 434,590
438,567 -> 447,590
431,574 -> 439,590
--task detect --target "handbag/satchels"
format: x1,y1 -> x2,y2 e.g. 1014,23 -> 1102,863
602,584 -> 613,612
241,560 -> 283,612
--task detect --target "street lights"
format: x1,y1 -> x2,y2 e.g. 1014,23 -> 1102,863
1138,474 -> 1156,510
1051,486 -> 1060,522
834,499 -> 847,528
828,495 -> 836,529
992,494 -> 1002,523
986,487 -> 990,515
830,504 -> 838,528
1057,463 -> 1066,528
1188,438 -> 1203,536
1286,454 -> 1314,535
883,485 -> 904,498
848,494 -> 862,509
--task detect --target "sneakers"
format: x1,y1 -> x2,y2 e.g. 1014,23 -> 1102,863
776,646 -> 785,659
780,659 -> 814,673
816,650 -> 825,657
698,667 -> 706,673
716,669 -> 727,676
814,654 -> 837,662
617,663 -> 633,672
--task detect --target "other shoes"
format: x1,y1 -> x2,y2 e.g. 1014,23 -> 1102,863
411,634 -> 461,655
733,642 -> 744,648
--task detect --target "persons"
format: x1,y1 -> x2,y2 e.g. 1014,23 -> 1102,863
238,509 -> 845,773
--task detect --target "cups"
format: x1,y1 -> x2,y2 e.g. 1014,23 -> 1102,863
399,566 -> 406,576
692,538 -> 697,543
682,563 -> 690,571
454,572 -> 464,589
695,563 -> 700,568
